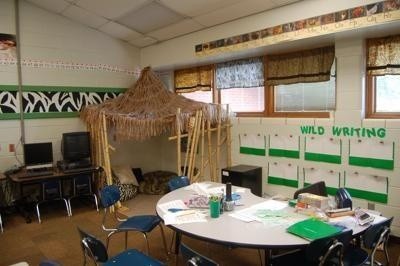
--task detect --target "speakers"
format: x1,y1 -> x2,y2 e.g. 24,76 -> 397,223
222,164 -> 262,197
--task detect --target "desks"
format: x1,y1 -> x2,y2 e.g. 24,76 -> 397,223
6,160 -> 105,224
155,180 -> 375,266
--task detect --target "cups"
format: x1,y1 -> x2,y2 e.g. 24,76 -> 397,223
210,201 -> 220,218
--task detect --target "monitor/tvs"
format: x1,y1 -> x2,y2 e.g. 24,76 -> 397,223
61,132 -> 90,161
23,142 -> 53,174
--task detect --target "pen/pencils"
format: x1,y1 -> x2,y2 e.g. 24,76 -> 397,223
210,195 -> 222,201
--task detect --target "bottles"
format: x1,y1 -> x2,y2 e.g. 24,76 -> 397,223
297,194 -> 306,219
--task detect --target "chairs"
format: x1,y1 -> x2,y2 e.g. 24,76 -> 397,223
35,173 -> 99,225
265,181 -> 395,266
77,175 -> 219,266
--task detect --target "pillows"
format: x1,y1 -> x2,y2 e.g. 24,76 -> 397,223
112,167 -> 140,186
